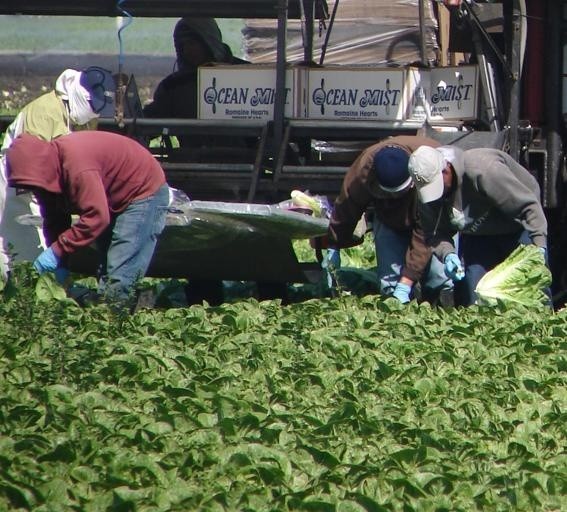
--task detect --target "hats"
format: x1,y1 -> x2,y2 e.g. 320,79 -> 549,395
87,66 -> 116,115
16,187 -> 32,195
408,145 -> 444,204
375,146 -> 412,192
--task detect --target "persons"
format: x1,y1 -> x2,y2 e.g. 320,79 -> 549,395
4,132 -> 169,313
320,134 -> 460,313
407,145 -> 551,312
139,17 -> 293,307
4,64 -> 115,282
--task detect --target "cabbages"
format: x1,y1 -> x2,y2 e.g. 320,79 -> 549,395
474,243 -> 553,315
291,189 -> 321,217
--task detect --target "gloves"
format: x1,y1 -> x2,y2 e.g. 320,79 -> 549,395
33,248 -> 58,273
540,248 -> 545,253
392,283 -> 411,304
444,255 -> 465,281
321,248 -> 341,270
